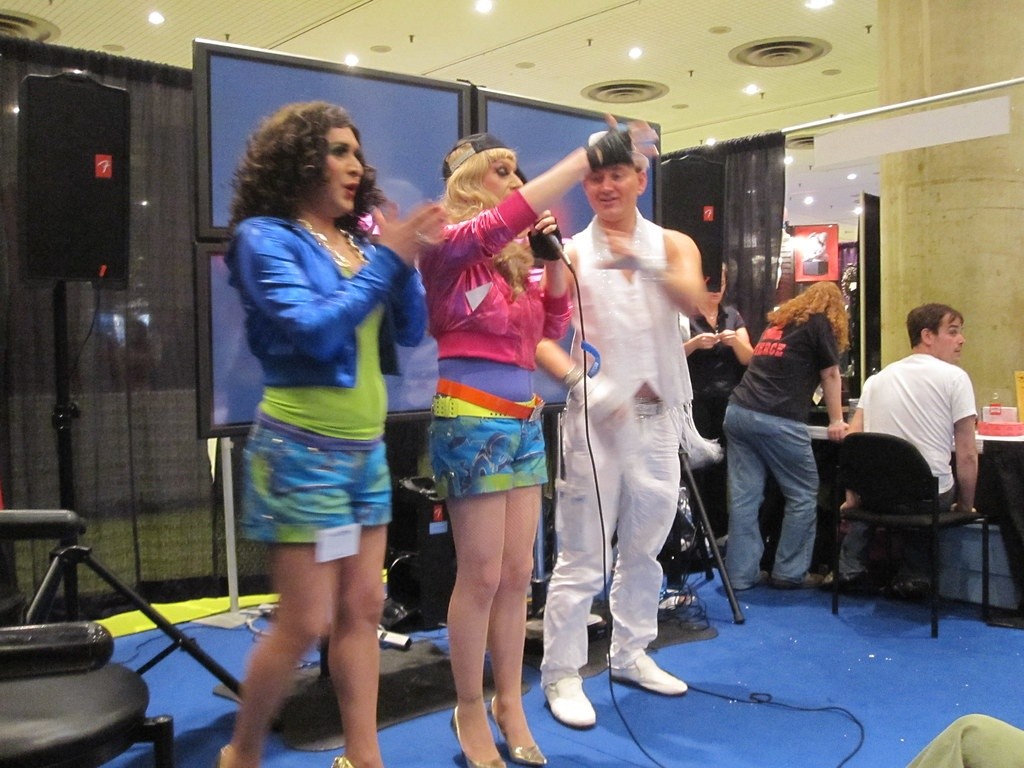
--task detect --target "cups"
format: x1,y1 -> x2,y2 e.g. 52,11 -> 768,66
848,398 -> 859,424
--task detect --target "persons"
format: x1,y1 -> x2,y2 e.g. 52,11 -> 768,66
539,128 -> 706,728
838,301 -> 978,594
723,280 -> 851,591
680,261 -> 753,560
419,110 -> 658,768
218,98 -> 449,768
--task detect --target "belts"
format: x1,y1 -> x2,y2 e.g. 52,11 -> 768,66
432,378 -> 545,423
634,401 -> 660,415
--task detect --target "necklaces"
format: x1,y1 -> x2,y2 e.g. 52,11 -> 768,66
700,306 -> 721,336
298,218 -> 370,274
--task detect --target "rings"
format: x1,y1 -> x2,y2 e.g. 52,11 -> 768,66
416,230 -> 424,238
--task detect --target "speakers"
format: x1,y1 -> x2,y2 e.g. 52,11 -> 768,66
387,476 -> 458,633
662,154 -> 724,292
20,70 -> 131,291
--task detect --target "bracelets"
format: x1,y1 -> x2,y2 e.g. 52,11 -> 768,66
830,418 -> 844,424
562,365 -> 586,386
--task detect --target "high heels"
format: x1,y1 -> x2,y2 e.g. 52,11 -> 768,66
451,706 -> 506,768
491,696 -> 547,765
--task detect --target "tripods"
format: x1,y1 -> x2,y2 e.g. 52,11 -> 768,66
22,283 -> 283,736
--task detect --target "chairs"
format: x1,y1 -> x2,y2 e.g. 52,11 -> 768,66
830,430 -> 990,638
0,509 -> 176,768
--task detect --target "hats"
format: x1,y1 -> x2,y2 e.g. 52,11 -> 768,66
442,132 -> 510,181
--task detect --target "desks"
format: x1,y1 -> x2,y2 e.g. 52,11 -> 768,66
807,418 -> 1024,627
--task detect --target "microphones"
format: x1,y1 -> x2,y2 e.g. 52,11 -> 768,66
543,234 -> 572,269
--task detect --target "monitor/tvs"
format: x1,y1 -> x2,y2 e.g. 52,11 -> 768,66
191,37 -> 663,437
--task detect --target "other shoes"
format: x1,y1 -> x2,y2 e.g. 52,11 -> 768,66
611,654 -> 687,694
545,676 -> 596,727
774,572 -> 826,587
759,571 -> 768,579
837,572 -> 870,595
886,584 -> 924,601
